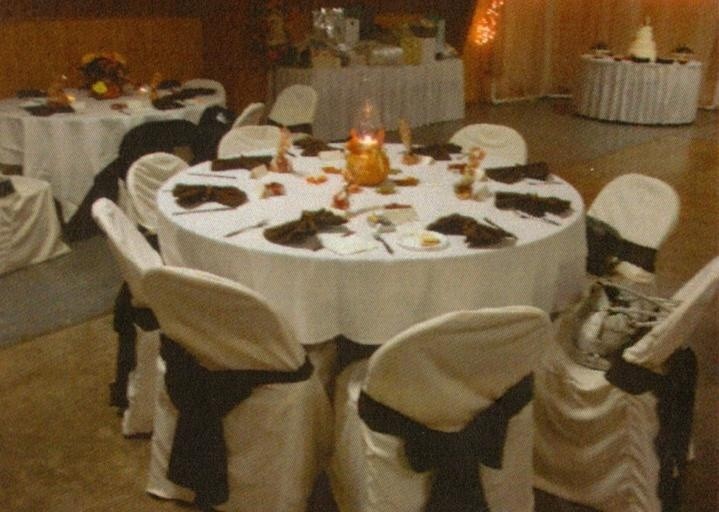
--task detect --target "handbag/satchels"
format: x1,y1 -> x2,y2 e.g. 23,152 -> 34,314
585,214 -> 623,276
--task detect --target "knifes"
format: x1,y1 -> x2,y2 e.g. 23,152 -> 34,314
171,206 -> 234,215
483,217 -> 518,239
187,173 -> 236,179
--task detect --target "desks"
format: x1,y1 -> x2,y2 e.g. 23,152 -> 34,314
156,150 -> 592,341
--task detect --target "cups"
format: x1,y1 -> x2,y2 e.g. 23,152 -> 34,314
595,49 -> 603,57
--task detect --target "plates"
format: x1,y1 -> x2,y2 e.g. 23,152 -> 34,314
398,228 -> 447,252
20,101 -> 44,109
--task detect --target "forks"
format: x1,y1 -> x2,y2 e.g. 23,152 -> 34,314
372,231 -> 393,254
223,221 -> 267,239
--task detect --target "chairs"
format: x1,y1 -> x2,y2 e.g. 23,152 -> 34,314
0,78 -> 531,275
139,265 -> 332,510
585,171 -> 682,301
533,255 -> 718,510
91,193 -> 163,442
332,303 -> 552,510
